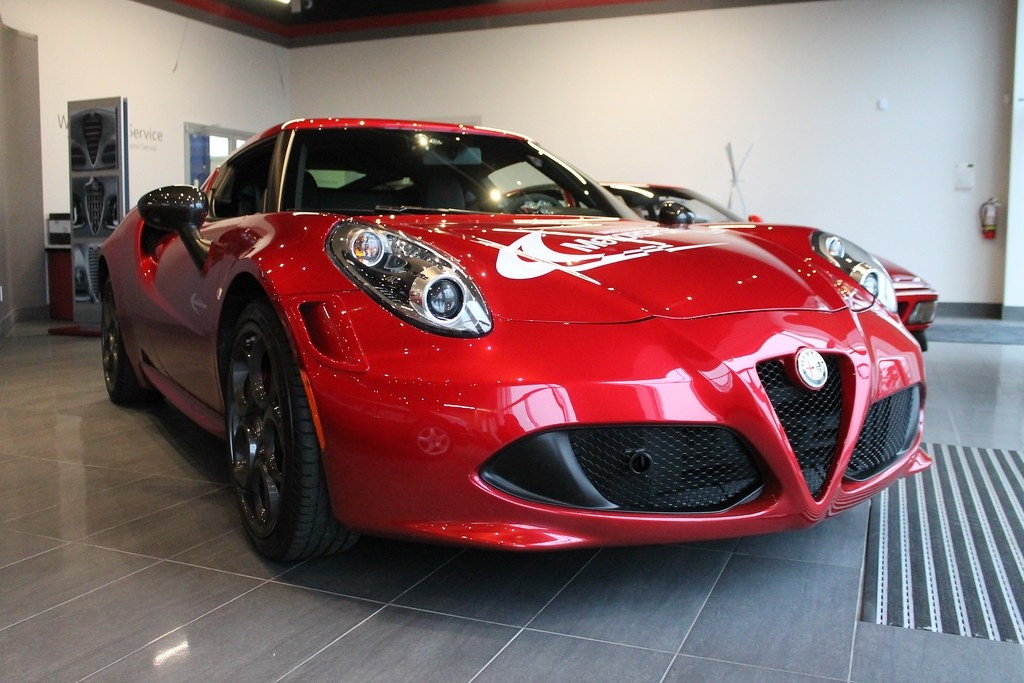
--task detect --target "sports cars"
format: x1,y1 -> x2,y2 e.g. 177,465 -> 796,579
496,182 -> 938,352
97,118 -> 934,563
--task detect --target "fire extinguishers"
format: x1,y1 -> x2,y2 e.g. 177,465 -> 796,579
983,197 -> 1000,239
